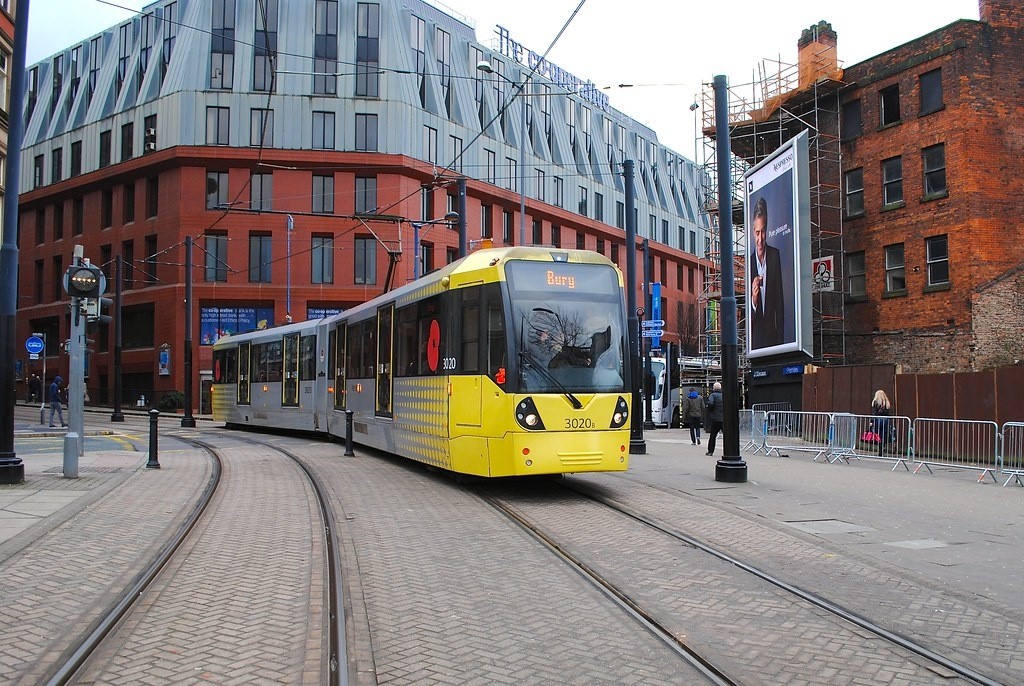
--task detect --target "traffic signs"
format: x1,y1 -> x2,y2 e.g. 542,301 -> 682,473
642,330 -> 665,337
642,320 -> 665,327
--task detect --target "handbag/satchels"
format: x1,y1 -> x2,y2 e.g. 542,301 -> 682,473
861,426 -> 880,445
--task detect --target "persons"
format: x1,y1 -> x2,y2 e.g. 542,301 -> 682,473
335,369 -> 343,405
239,375 -> 247,402
285,372 -> 295,404
28,374 -> 38,404
705,381 -> 723,456
750,197 -> 784,350
48,376 -> 68,427
869,390 -> 890,457
527,328 -> 561,392
64,384 -> 68,408
684,388 -> 705,445
378,364 -> 389,414
84,391 -> 86,396
739,395 -> 743,429
227,370 -> 234,382
552,330 -> 591,389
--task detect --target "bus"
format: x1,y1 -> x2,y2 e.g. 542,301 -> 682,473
210,246 -> 632,479
640,355 -> 721,428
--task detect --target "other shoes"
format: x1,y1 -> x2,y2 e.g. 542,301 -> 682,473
49,425 -> 55,427
697,437 -> 700,445
62,424 -> 67,427
691,443 -> 696,445
706,452 -> 713,456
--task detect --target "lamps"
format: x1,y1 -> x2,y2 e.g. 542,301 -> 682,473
476,61 -> 495,74
67,265 -> 101,299
444,212 -> 460,221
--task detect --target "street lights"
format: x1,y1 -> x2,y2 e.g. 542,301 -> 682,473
477,59 -> 527,246
410,210 -> 459,282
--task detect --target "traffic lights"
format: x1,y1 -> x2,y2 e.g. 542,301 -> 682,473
98,296 -> 113,324
66,264 -> 102,299
